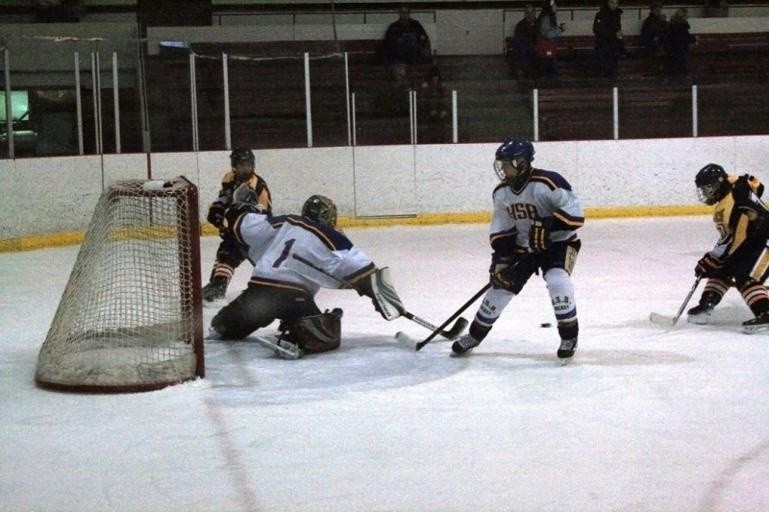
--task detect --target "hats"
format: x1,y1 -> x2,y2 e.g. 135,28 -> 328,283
650,1 -> 664,8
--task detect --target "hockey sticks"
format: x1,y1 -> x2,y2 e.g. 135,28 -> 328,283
397,259 -> 523,351
650,238 -> 722,327
290,253 -> 466,341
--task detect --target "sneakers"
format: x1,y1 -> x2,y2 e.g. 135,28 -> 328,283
742,297 -> 769,333
451,333 -> 481,355
686,291 -> 715,324
556,337 -> 579,365
273,334 -> 304,360
201,282 -> 226,303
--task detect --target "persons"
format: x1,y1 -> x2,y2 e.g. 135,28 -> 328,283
452,138 -> 586,367
372,60 -> 412,118
206,194 -> 403,360
417,68 -> 449,131
516,1 -> 699,85
384,5 -> 429,65
201,147 -> 273,303
688,163 -> 769,336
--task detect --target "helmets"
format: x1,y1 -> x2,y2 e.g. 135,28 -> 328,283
230,147 -> 255,179
695,163 -> 729,205
301,194 -> 338,230
494,138 -> 536,181
